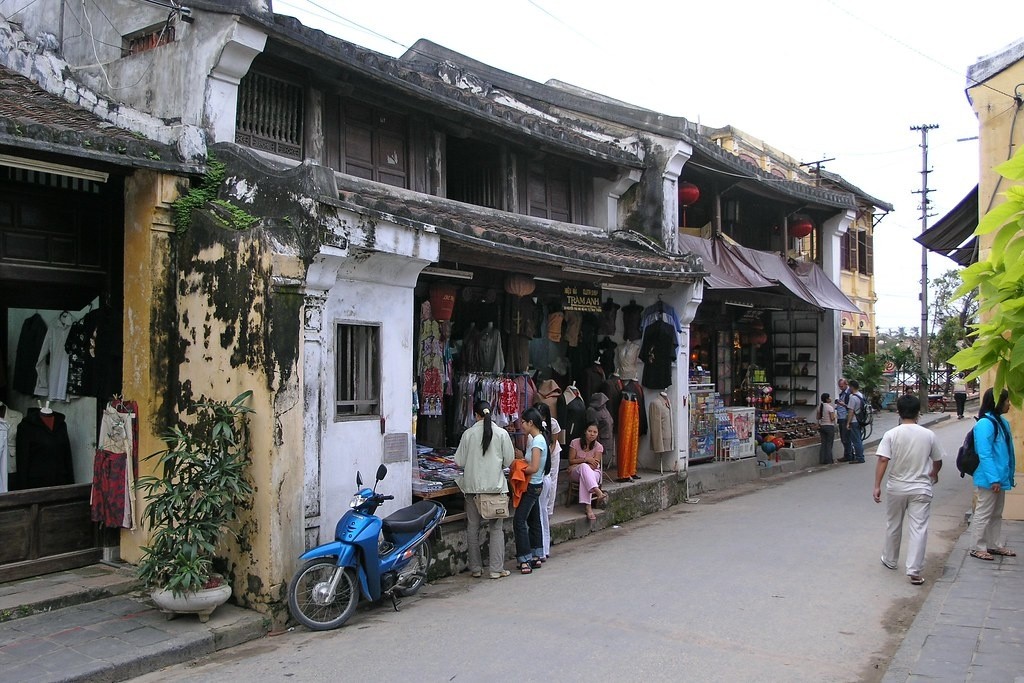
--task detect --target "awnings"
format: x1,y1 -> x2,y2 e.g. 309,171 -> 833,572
678,233 -> 867,315
912,182 -> 979,268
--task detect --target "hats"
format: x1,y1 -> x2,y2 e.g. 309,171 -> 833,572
846,381 -> 859,388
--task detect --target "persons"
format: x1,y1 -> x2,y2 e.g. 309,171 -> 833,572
833,379 -> 853,462
951,372 -> 967,419
510,401 -> 562,574
617,391 -> 641,482
569,423 -> 606,519
873,395 -> 947,583
649,388 -> 674,452
896,386 -> 919,425
970,388 -> 1017,560
816,393 -> 836,464
846,381 -> 865,464
455,400 -> 515,578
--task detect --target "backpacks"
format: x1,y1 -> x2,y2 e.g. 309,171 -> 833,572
956,415 -> 999,478
853,394 -> 873,427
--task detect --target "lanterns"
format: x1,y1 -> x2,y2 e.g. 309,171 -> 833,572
791,218 -> 812,256
678,180 -> 699,226
504,273 -> 535,296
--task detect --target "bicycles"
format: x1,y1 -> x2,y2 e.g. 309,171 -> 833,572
851,423 -> 873,446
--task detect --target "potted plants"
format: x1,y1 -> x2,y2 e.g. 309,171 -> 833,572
128,389 -> 257,625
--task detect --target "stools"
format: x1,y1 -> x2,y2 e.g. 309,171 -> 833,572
565,482 -> 602,511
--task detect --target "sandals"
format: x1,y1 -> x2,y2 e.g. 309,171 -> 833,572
970,550 -> 994,561
597,495 -> 606,502
584,508 -> 597,520
521,556 -> 541,574
987,547 -> 1016,556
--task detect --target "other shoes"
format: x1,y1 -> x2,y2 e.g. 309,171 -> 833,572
850,460 -> 861,464
472,569 -> 484,578
958,415 -> 964,419
541,555 -> 550,562
881,555 -> 898,570
489,570 -> 511,579
821,461 -> 834,464
910,577 -> 924,585
837,458 -> 850,462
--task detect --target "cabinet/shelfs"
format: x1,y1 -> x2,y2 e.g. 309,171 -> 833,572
689,310 -> 825,448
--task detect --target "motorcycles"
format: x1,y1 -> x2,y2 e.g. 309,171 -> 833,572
288,463 -> 448,631
928,395 -> 949,413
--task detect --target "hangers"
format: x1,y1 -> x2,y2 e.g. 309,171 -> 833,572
32,303 -> 97,314
116,393 -> 128,414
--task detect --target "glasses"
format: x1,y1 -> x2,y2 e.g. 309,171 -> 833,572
520,420 -> 526,425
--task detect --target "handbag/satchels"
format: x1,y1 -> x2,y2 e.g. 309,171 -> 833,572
954,378 -> 966,391
473,494 -> 510,520
